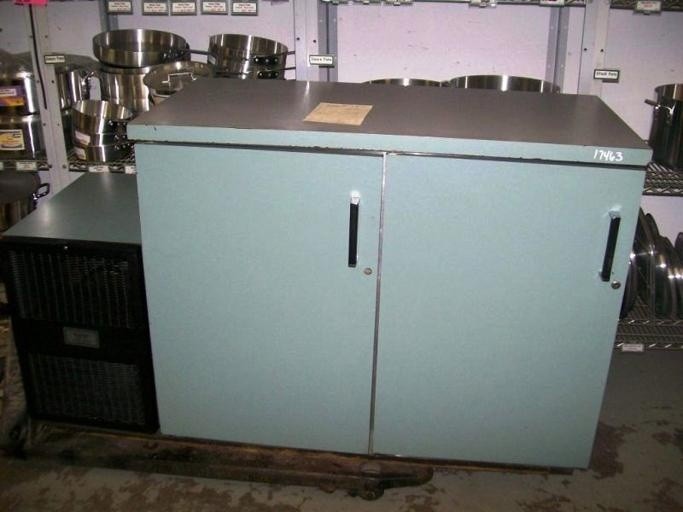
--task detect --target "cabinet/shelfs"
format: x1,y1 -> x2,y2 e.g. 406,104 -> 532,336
126,77 -> 654,467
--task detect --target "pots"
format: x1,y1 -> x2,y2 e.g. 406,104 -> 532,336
644,84 -> 682,170
55,26 -> 296,162
